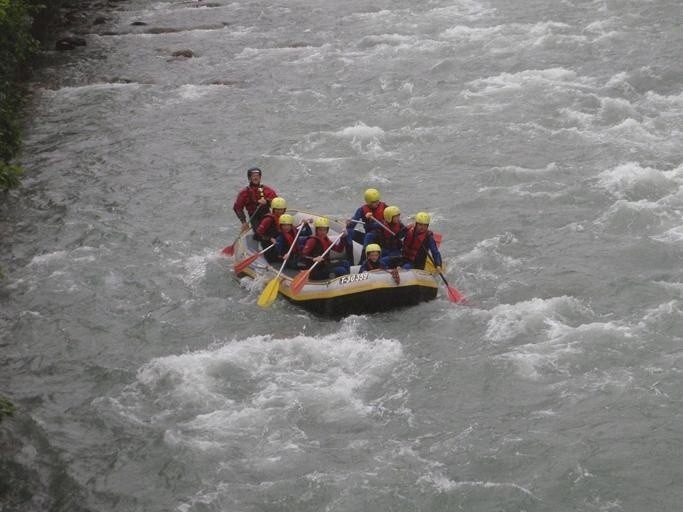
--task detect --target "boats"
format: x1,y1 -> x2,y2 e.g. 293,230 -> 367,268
230,217 -> 442,322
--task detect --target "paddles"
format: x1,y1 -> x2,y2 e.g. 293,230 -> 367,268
223,204 -> 260,255
365,212 -> 463,303
257,222 -> 305,307
234,219 -> 311,273
291,232 -> 345,296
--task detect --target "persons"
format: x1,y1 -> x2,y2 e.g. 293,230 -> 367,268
346,188 -> 388,266
389,212 -> 442,273
300,217 -> 349,280
232,168 -> 277,231
273,214 -> 313,269
254,197 -> 287,264
365,205 -> 406,268
358,244 -> 388,272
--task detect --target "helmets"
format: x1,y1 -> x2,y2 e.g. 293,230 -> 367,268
366,244 -> 381,259
315,218 -> 331,235
278,213 -> 292,228
365,188 -> 381,206
271,196 -> 287,213
384,206 -> 403,223
414,212 -> 431,230
248,167 -> 261,180
